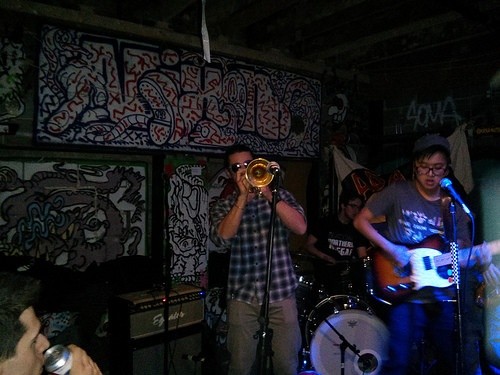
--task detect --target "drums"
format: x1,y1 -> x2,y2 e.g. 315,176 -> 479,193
305,294 -> 391,375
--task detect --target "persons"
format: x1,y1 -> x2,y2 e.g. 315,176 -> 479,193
353,126 -> 492,375
304,192 -> 373,315
209,144 -> 306,374
0,263 -> 104,375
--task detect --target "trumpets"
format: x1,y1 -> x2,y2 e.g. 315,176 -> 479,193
245,158 -> 274,197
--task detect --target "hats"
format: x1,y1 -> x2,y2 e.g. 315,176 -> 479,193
413,134 -> 450,154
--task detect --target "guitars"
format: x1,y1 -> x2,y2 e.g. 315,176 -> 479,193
373,232 -> 500,300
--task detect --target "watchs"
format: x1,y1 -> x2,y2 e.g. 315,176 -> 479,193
268,194 -> 282,208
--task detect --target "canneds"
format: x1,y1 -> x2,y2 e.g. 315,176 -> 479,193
43,345 -> 73,375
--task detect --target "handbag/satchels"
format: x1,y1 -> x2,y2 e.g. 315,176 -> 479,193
100,254 -> 166,295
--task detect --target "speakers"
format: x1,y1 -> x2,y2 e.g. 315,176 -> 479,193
118,282 -> 207,375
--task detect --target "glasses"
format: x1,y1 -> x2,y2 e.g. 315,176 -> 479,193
346,202 -> 363,212
231,160 -> 253,173
418,163 -> 450,177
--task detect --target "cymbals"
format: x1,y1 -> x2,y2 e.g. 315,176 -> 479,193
289,250 -> 320,259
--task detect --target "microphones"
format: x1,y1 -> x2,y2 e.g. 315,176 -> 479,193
363,359 -> 372,370
438,178 -> 473,219
181,353 -> 206,362
267,161 -> 282,176
